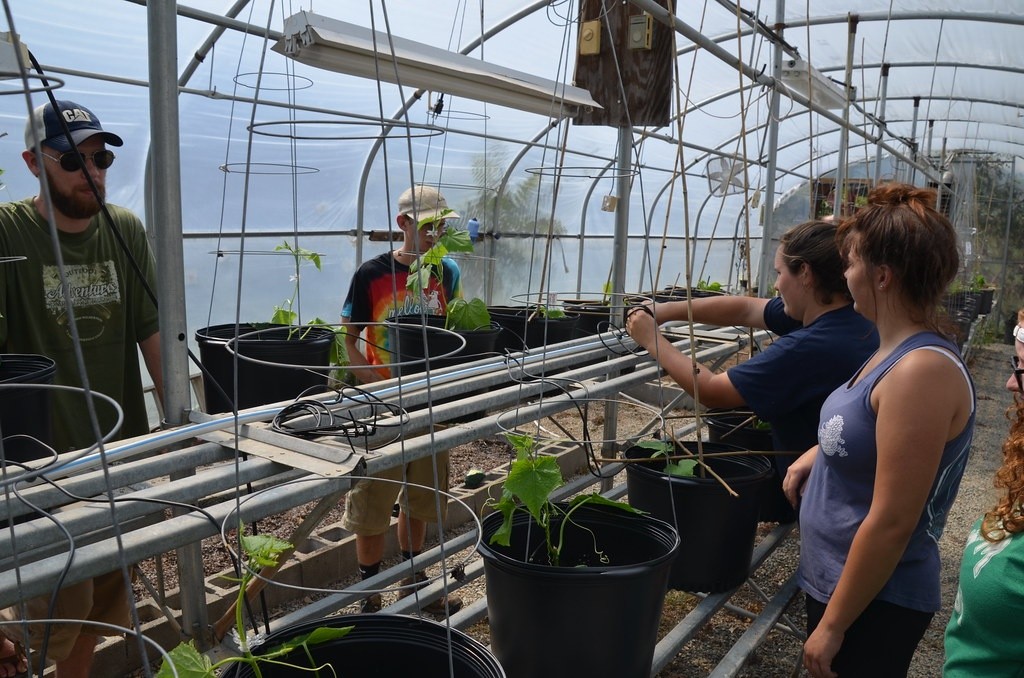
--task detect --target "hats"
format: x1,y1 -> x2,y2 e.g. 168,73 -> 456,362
399,184 -> 460,223
24,99 -> 123,152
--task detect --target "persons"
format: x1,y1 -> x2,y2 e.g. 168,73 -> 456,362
0,99 -> 170,678
341,182 -> 464,617
781,182 -> 981,678
624,219 -> 882,516
941,307 -> 1024,678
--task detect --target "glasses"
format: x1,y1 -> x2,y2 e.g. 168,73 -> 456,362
42,149 -> 115,172
1011,355 -> 1024,392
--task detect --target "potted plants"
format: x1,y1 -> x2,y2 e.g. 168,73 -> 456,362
474,429 -> 681,678
942,278 -> 970,309
156,516 -> 507,678
194,238 -> 351,415
664,275 -> 731,298
562,280 -> 643,336
486,306 -> 581,353
618,440 -> 772,594
966,275 -> 996,315
702,408 -> 798,524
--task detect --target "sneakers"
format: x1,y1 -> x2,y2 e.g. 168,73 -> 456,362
398,579 -> 463,615
361,592 -> 382,613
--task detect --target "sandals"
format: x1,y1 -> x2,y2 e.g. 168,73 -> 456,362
0,650 -> 33,678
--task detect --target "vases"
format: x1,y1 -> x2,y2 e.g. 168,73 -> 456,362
383,207 -> 505,379
642,292 -> 688,303
954,317 -> 971,342
0,352 -> 58,466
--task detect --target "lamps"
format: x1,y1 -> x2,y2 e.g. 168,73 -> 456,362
270,11 -> 604,120
781,59 -> 849,110
